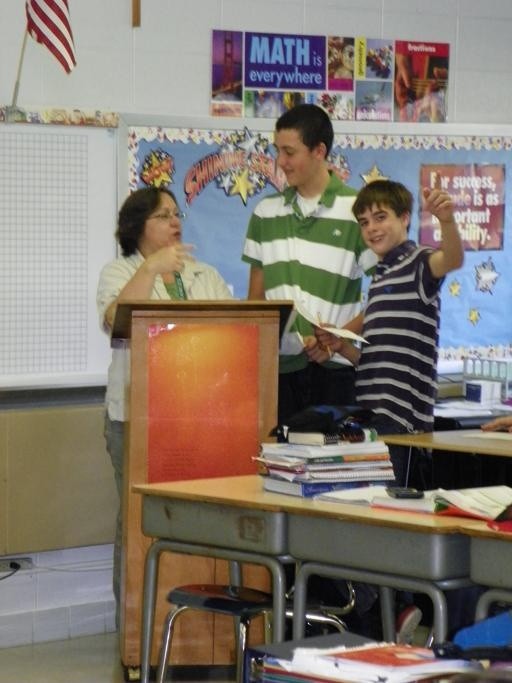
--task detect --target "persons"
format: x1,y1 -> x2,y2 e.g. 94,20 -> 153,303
93,182 -> 236,633
238,102 -> 378,638
313,178 -> 470,649
481,413 -> 512,435
393,39 -> 450,121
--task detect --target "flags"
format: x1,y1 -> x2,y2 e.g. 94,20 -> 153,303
24,0 -> 77,74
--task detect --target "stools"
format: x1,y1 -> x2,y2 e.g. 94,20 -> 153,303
158,584 -> 274,681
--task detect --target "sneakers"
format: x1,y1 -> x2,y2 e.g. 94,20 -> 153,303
396,604 -> 423,647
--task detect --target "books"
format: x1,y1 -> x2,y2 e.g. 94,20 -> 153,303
251,421 -> 511,522
247,632 -> 476,682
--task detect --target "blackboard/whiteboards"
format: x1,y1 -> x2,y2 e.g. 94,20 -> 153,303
0,109 -> 124,393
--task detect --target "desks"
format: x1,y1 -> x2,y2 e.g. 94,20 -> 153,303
132,473 -> 283,682
282,490 -> 459,656
460,516 -> 511,624
376,432 -> 512,492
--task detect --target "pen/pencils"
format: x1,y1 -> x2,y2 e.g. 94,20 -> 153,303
317,312 -> 332,360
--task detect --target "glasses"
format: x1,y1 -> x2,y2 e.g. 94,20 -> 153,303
148,210 -> 186,221
